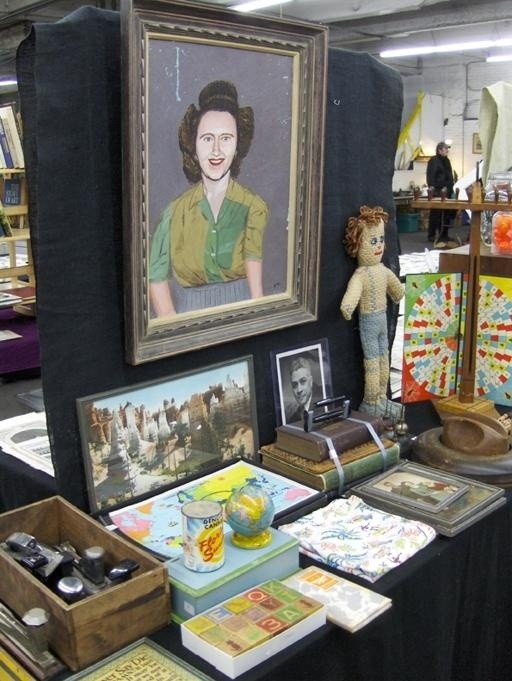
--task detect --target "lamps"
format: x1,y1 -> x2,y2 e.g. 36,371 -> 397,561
485,51 -> 512,63
381,34 -> 512,59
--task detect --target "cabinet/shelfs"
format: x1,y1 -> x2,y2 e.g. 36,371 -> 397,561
0,168 -> 37,288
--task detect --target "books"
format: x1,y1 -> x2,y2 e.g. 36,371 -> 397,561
0,103 -> 37,309
274,408 -> 386,463
258,444 -> 400,495
279,565 -> 391,634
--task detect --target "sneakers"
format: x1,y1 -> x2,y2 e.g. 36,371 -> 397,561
428,235 -> 435,242
439,237 -> 454,241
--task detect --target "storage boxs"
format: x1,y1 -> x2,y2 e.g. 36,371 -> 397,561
397,213 -> 419,233
0,494 -> 173,673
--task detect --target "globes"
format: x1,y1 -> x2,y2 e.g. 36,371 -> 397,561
225,483 -> 275,549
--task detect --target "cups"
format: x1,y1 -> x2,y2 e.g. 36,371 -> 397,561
181,500 -> 225,573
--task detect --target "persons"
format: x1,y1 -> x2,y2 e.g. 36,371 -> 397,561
288,357 -> 324,424
340,205 -> 406,419
426,141 -> 455,241
384,479 -> 459,506
147,81 -> 268,317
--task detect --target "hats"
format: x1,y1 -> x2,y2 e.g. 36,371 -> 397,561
199,81 -> 238,110
436,142 -> 451,149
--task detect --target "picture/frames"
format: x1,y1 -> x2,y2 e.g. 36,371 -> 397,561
472,132 -> 482,154
118,0 -> 327,364
75,354 -> 261,520
351,460 -> 507,537
65,636 -> 216,681
270,338 -> 336,426
